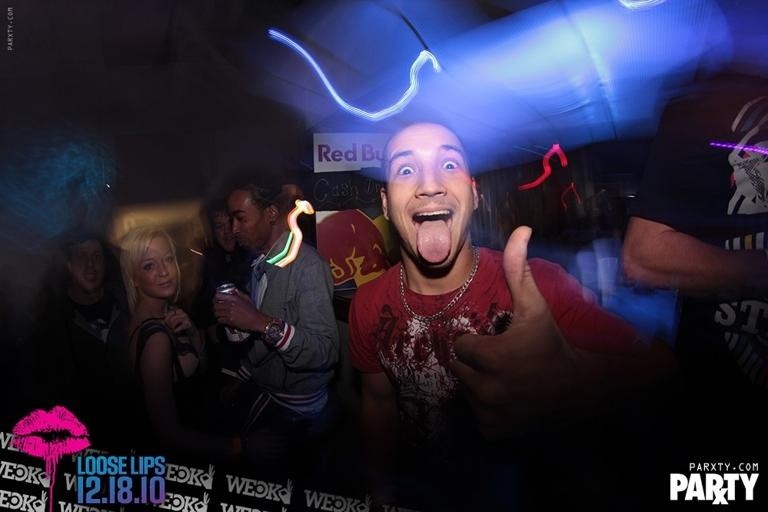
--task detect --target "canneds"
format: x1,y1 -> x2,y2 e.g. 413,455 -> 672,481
217,283 -> 253,345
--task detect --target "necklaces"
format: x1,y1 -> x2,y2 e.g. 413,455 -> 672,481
399,245 -> 479,320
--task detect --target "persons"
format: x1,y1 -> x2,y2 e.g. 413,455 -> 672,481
36,179 -> 341,512
621,0 -> 768,512
346,122 -> 676,512
